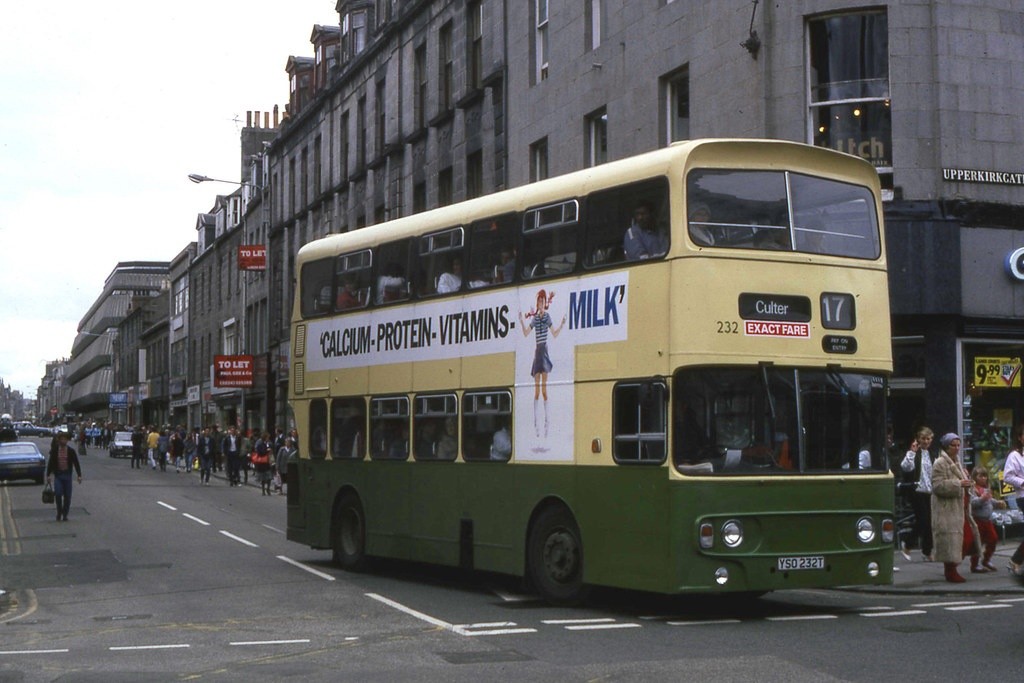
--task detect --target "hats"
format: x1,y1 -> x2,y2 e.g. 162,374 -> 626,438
940,432 -> 961,447
688,202 -> 711,220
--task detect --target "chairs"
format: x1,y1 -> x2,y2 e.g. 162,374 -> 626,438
313,434 -> 494,461
312,263 -> 545,315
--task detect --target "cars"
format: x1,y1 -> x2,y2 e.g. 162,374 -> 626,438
11,420 -> 51,438
108,431 -> 134,457
0,442 -> 47,483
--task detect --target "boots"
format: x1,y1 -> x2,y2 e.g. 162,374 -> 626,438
944,563 -> 966,582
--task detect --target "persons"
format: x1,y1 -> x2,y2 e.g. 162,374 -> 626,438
519,290 -> 566,438
36,420 -> 297,495
900,428 -> 937,560
336,242 -> 531,308
646,395 -> 715,464
321,408 -> 511,459
622,201 -> 825,259
1004,428 -> 1024,576
7,425 -> 16,442
971,467 -> 1006,570
858,425 -> 892,470
47,434 -> 82,521
933,433 -> 982,581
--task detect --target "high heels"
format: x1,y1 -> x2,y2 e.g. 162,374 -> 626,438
1007,559 -> 1021,576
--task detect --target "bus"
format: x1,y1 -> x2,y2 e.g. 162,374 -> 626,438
283,138 -> 896,608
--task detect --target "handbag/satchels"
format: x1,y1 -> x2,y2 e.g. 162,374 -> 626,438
273,470 -> 281,490
251,451 -> 269,464
42,482 -> 54,504
895,481 -> 914,499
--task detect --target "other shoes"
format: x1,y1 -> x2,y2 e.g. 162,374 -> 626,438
63,517 -> 67,522
57,514 -> 62,522
920,553 -> 933,562
131,460 -> 272,497
971,561 -> 988,572
982,560 -> 996,571
901,541 -> 912,564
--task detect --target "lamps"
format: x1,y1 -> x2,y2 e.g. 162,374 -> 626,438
188,173 -> 262,192
